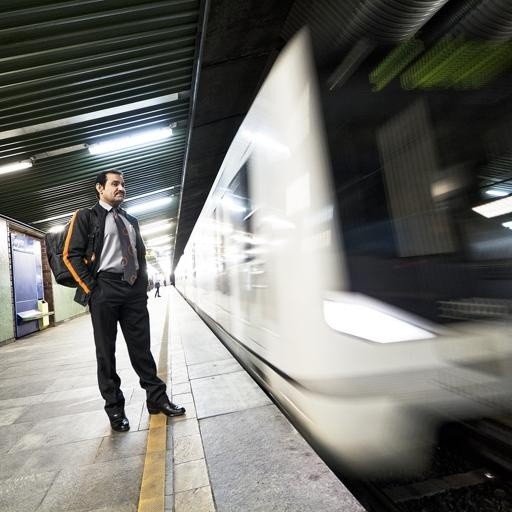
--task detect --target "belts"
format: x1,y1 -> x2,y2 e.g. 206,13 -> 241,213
99,271 -> 124,281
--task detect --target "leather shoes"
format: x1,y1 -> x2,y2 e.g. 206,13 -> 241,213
105,399 -> 130,432
146,399 -> 186,417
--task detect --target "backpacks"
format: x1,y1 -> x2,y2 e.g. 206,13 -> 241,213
44,207 -> 101,288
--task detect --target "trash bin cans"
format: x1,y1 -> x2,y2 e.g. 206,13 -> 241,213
38,299 -> 50,331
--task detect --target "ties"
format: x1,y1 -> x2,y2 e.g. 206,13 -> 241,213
112,209 -> 137,286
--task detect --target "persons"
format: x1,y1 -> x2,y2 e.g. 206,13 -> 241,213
62,170 -> 186,432
155,281 -> 161,298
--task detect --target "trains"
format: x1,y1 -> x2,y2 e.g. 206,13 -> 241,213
174,1 -> 512,484
